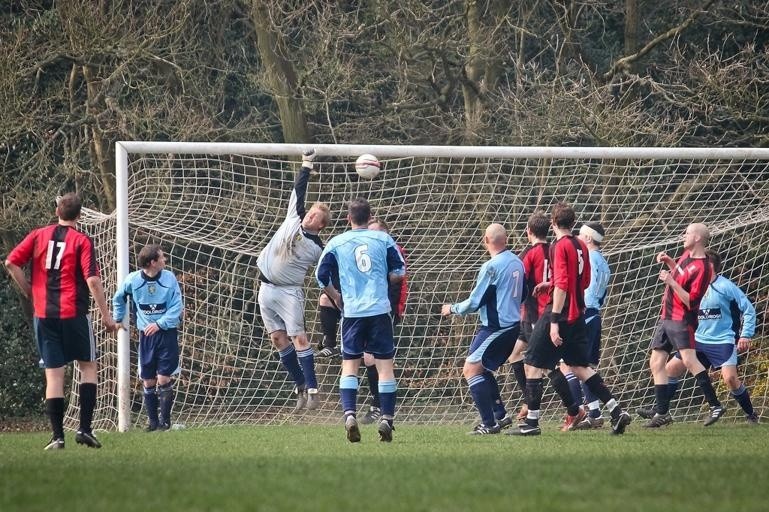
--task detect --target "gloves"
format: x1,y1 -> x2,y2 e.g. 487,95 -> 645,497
302,148 -> 317,161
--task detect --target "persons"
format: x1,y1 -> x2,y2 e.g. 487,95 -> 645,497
314,219 -> 407,423
634,249 -> 758,423
255,149 -> 332,414
642,223 -> 727,427
112,244 -> 184,433
314,199 -> 406,444
559,222 -> 611,430
442,223 -> 525,434
508,211 -> 587,431
4,194 -> 116,452
502,202 -> 631,435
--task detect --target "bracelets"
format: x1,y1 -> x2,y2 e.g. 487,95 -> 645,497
550,311 -> 561,324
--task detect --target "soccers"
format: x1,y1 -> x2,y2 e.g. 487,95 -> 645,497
355,154 -> 380,179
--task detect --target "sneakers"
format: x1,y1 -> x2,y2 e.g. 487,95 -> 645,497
468,423 -> 500,436
159,422 -> 171,432
378,419 -> 396,443
143,424 -> 158,433
637,408 -> 656,420
314,348 -> 339,359
497,413 -> 513,429
745,411 -> 757,423
359,406 -> 381,425
561,409 -> 588,432
345,415 -> 361,443
704,405 -> 726,426
573,416 -> 604,430
294,387 -> 306,410
610,411 -> 632,435
307,388 -> 320,410
75,428 -> 102,449
642,413 -> 673,428
45,437 -> 65,451
504,423 -> 542,436
